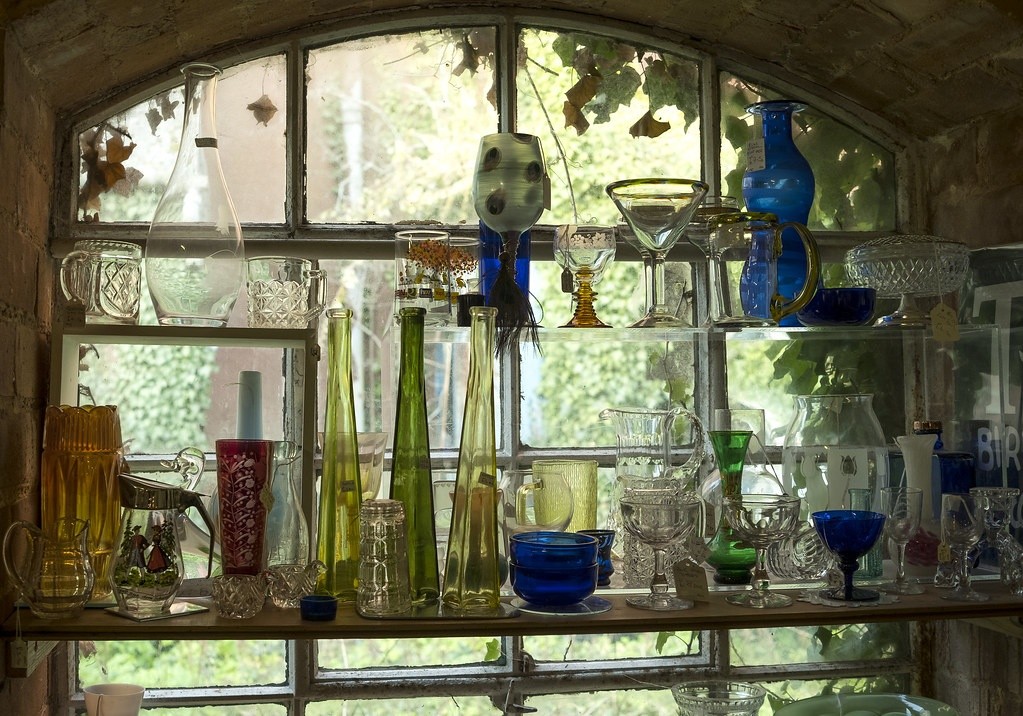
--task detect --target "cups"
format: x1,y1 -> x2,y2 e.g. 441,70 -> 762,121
245,255 -> 327,330
44,402 -> 310,618
355,498 -> 411,619
449,236 -> 479,325
391,229 -> 452,328
82,683 -> 145,716
706,213 -> 822,328
668,680 -> 765,716
516,459 -> 599,532
59,239 -> 143,326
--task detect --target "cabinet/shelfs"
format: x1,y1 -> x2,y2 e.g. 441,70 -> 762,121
2,319 -> 1023,639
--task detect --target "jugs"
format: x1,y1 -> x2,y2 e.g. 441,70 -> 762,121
3,516 -> 96,621
109,472 -> 216,619
598,406 -> 706,574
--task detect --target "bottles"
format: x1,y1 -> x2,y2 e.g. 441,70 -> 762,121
780,393 -> 889,580
703,429 -> 762,584
739,99 -> 816,327
319,307 -> 365,602
144,61 -> 246,328
846,487 -> 885,579
913,420 -> 980,569
389,307 -> 439,613
266,440 -> 309,570
887,435 -> 940,583
694,408 -> 788,543
443,306 -> 504,614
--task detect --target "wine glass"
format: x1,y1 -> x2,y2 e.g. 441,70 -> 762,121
607,176 -> 742,327
313,432 -> 1022,612
553,224 -> 616,329
474,132 -> 546,329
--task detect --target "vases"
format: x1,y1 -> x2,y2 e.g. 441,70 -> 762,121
145,61 -> 245,330
700,393 -> 979,584
208,307 -> 501,613
739,100 -> 822,327
41,405 -> 122,598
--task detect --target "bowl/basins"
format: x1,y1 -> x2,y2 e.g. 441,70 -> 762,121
794,288 -> 876,327
774,693 -> 959,716
508,560 -> 598,608
509,530 -> 599,570
